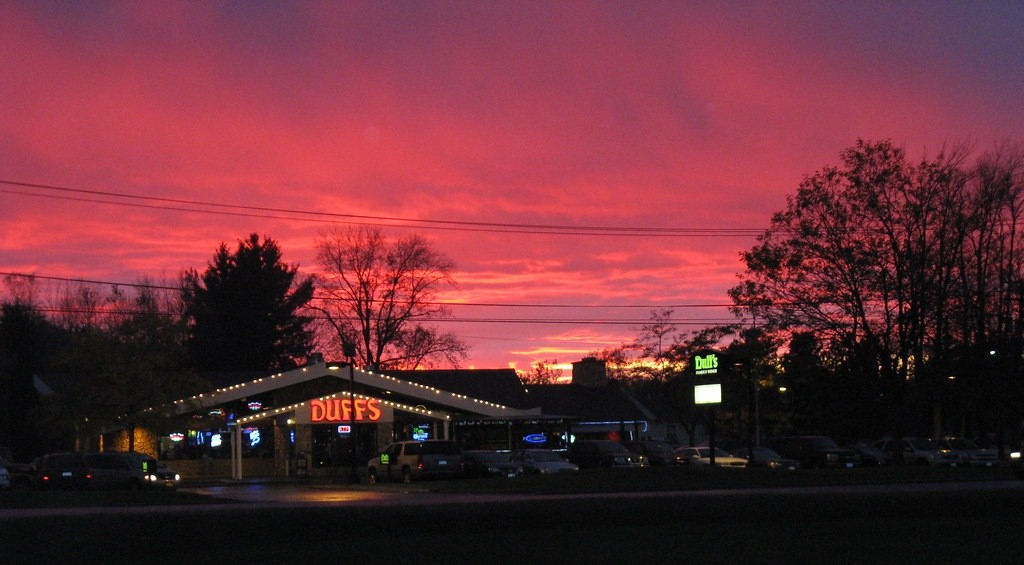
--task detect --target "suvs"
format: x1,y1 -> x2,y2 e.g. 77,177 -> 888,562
566,440 -> 651,471
770,435 -> 859,471
366,440 -> 466,486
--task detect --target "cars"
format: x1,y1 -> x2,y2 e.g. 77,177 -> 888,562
863,436 -> 965,468
954,433 -> 1019,466
35,451 -> 94,493
508,450 -> 579,477
732,447 -> 802,471
464,449 -> 526,478
675,445 -> 751,470
630,440 -> 692,466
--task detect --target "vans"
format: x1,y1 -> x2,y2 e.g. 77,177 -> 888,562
85,452 -> 180,491
